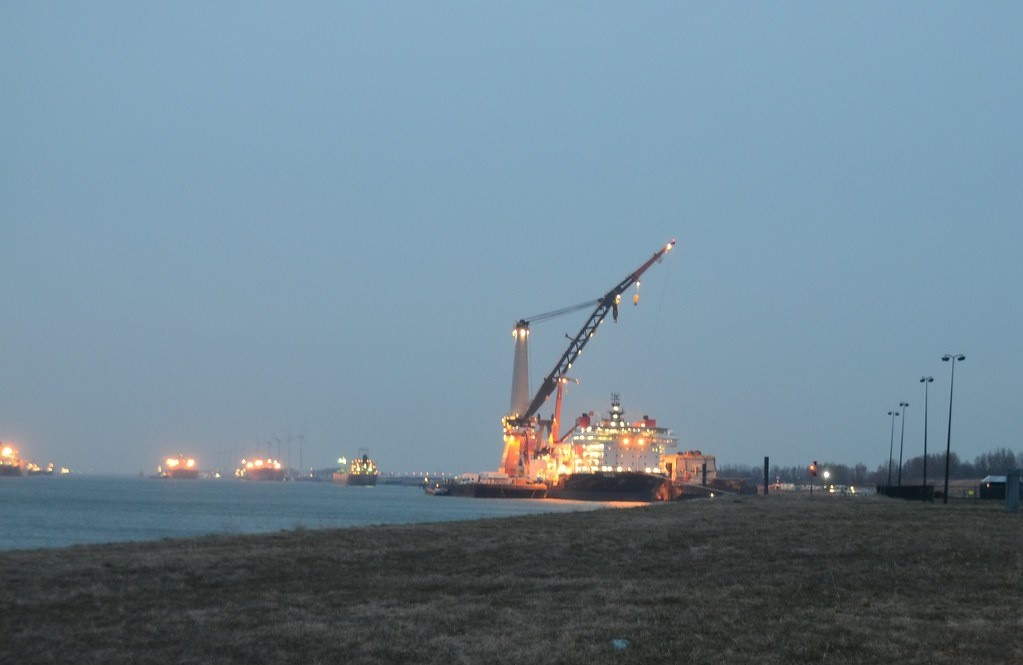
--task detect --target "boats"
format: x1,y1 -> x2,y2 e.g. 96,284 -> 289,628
156,454 -> 200,480
332,454 -> 379,486
424,483 -> 448,496
0,443 -> 25,478
235,457 -> 285,484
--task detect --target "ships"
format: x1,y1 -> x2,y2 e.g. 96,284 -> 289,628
443,388 -> 678,502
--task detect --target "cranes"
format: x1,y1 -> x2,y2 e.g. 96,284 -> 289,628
497,239 -> 676,480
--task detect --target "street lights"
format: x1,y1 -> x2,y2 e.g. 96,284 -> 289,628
810,466 -> 815,495
942,353 -> 966,504
898,402 -> 909,488
919,376 -> 934,502
887,411 -> 899,489
